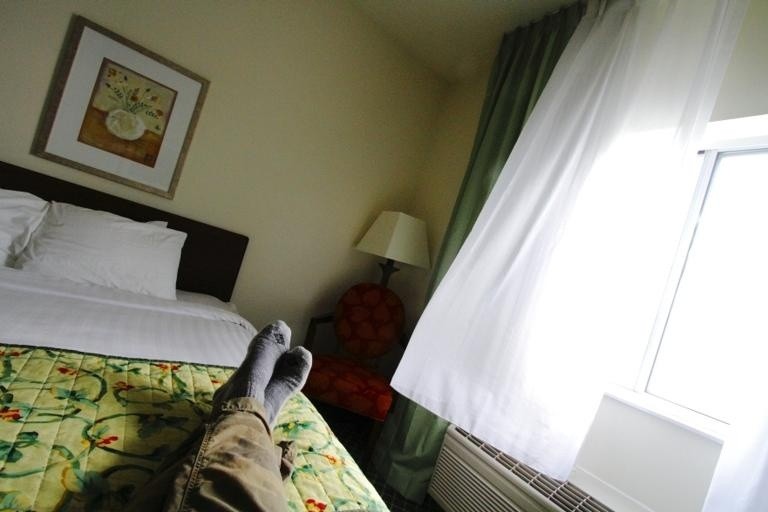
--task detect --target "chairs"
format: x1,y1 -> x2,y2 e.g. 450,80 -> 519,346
301,282 -> 409,450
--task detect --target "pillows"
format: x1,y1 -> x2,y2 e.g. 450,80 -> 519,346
1,188 -> 187,300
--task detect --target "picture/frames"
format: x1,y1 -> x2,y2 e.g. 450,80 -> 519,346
29,14 -> 212,202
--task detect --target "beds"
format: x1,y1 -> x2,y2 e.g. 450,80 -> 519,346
0,162 -> 392,512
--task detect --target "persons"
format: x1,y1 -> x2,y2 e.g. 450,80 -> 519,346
113,318 -> 315,512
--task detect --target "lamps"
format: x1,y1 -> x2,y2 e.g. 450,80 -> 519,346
357,209 -> 432,289
